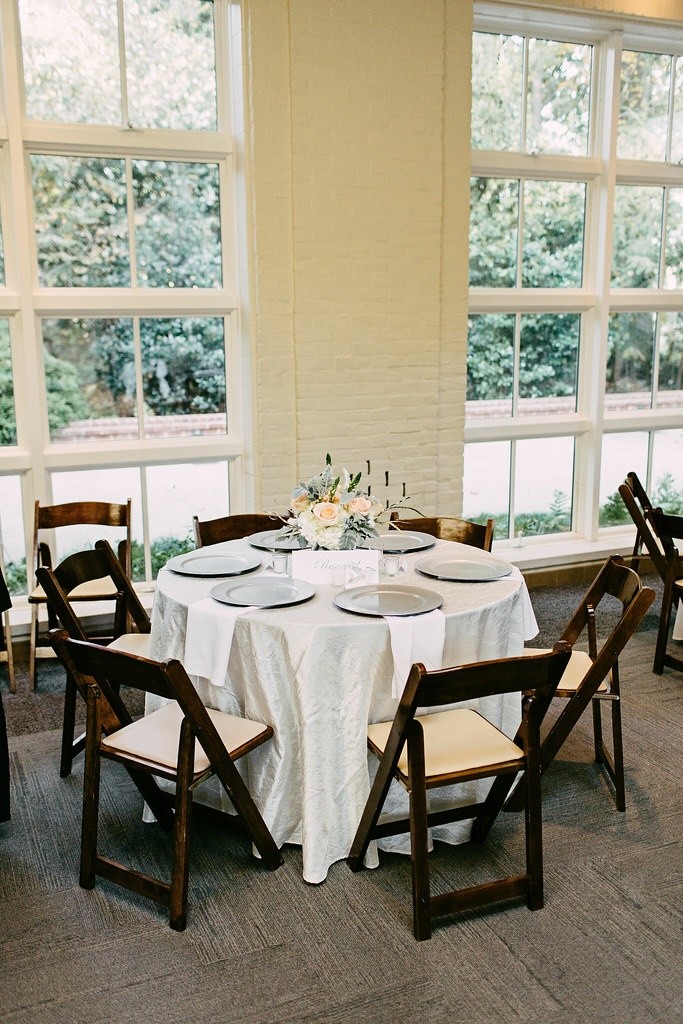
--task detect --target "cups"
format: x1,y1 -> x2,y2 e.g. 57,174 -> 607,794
271,552 -> 289,577
383,555 -> 401,578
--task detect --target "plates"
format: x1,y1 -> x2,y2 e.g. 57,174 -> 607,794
357,530 -> 436,551
334,584 -> 443,616
414,554 -> 513,581
165,549 -> 262,577
210,576 -> 318,607
246,529 -> 313,549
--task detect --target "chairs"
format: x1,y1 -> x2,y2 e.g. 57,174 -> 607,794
27,467 -> 683,941
